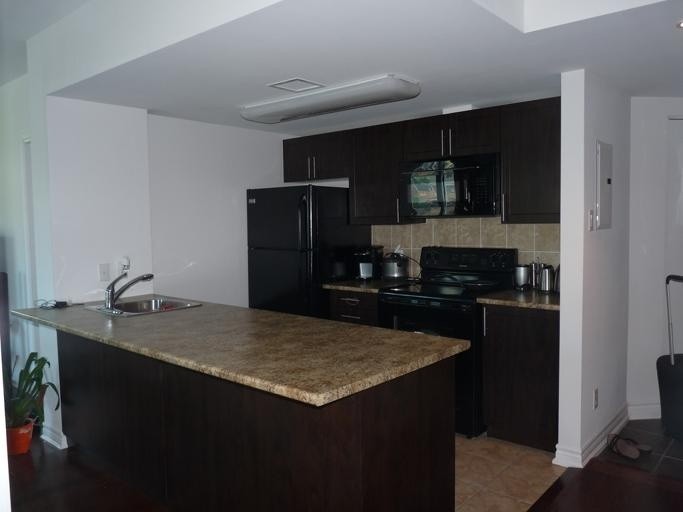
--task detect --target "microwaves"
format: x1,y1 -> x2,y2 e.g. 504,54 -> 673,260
397,154 -> 502,218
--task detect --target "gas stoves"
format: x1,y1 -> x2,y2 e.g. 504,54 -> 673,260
378,273 -> 518,312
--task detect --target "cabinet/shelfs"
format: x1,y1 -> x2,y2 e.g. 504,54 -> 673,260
348,122 -> 427,224
401,105 -> 499,164
283,129 -> 351,183
328,289 -> 378,327
499,95 -> 560,225
479,305 -> 560,454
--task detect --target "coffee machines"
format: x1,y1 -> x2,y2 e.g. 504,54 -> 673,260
354,245 -> 383,282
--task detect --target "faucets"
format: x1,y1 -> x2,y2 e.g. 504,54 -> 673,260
105,273 -> 154,309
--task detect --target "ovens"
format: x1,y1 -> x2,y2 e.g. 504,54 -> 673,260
376,301 -> 488,438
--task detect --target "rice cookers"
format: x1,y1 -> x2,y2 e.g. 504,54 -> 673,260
381,251 -> 408,282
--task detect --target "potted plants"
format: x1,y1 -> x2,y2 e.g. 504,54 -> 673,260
3,352 -> 60,456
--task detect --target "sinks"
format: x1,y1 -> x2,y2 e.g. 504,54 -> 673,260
86,300 -> 202,317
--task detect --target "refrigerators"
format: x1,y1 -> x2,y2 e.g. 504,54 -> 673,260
247,184 -> 372,319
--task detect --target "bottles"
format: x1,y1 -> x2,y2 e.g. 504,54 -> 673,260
514,262 -> 560,296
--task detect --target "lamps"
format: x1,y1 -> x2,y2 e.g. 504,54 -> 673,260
239,73 -> 421,124
